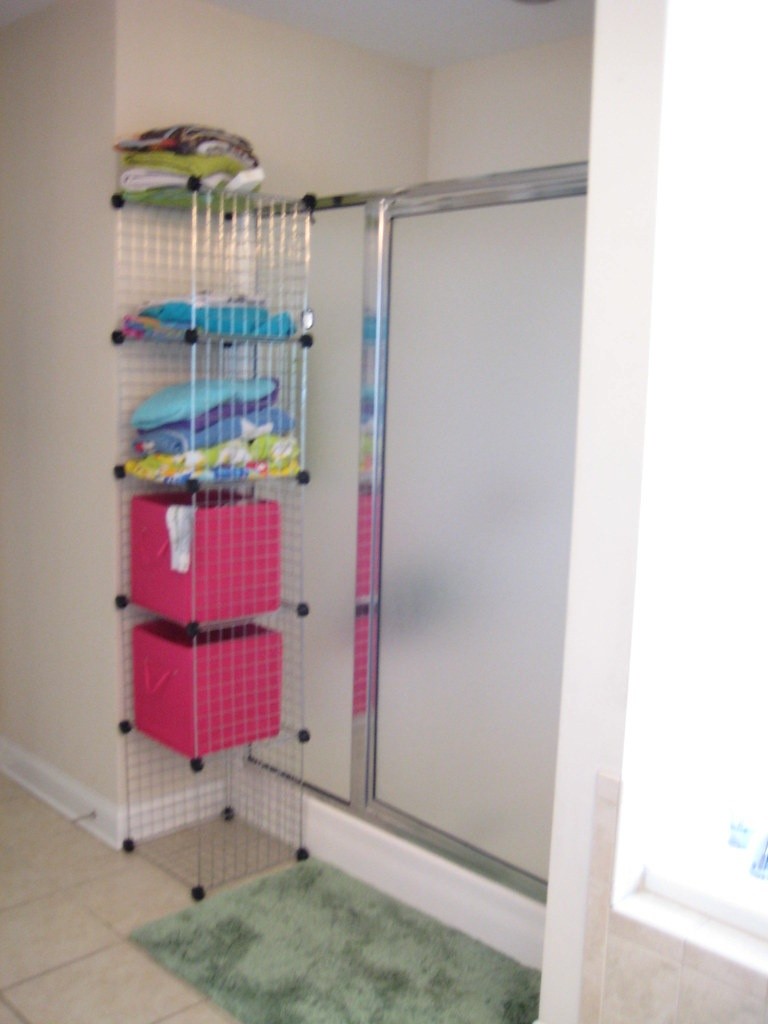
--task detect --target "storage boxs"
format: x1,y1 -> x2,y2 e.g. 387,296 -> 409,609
131,616 -> 284,761
127,490 -> 284,627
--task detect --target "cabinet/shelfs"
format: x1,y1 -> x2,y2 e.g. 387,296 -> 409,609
112,174 -> 319,901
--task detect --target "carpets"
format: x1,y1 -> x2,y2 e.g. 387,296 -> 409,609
128,860 -> 541,1024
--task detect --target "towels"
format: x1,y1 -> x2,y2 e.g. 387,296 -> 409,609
113,122 -> 264,194
125,374 -> 300,485
165,503 -> 198,574
118,291 -> 297,349
